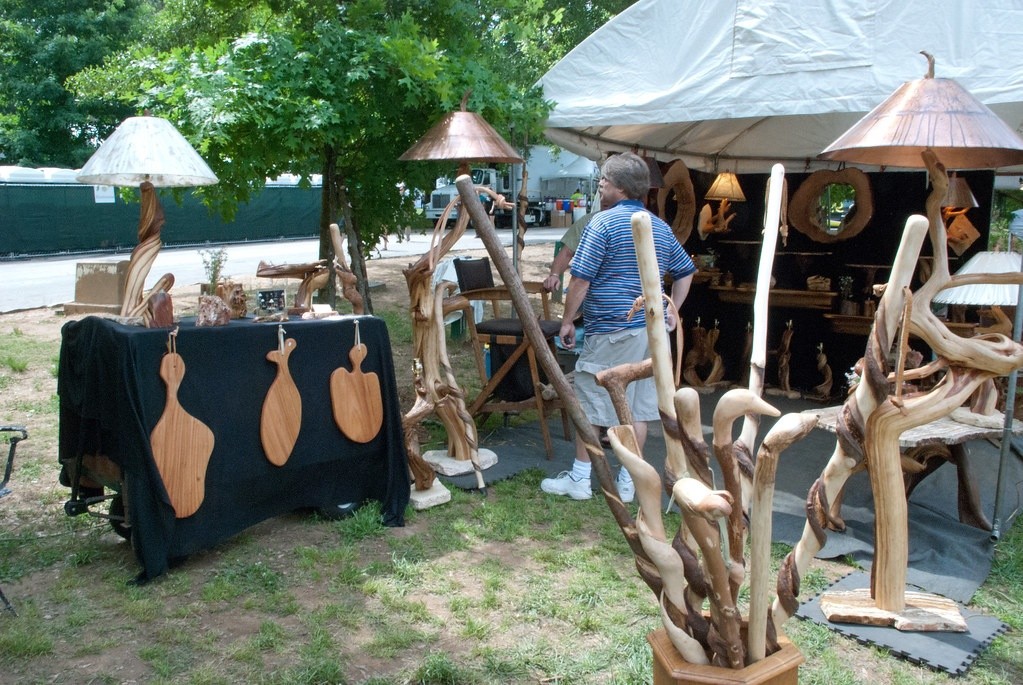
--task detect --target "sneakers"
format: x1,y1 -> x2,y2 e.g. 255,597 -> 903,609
613,477 -> 635,502
540,470 -> 593,500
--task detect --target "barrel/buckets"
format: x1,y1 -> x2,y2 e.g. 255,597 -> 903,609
577,198 -> 584,207
556,200 -> 574,212
573,207 -> 587,223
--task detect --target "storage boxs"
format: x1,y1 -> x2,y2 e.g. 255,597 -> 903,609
550,199 -> 574,227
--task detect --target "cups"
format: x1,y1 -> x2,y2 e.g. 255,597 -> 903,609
483,344 -> 490,378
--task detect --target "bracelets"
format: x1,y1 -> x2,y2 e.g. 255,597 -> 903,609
550,274 -> 560,278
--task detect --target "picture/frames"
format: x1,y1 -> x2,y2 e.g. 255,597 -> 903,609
257,289 -> 287,312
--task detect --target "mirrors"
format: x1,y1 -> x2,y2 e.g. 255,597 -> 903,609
787,167 -> 873,242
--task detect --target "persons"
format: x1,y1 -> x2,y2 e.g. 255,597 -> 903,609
540,152 -> 696,501
571,189 -> 584,207
543,200 -> 612,448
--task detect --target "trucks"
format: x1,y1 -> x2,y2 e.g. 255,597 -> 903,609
424,144 -> 603,229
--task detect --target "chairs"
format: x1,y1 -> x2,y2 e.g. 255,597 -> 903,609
455,255 -> 577,459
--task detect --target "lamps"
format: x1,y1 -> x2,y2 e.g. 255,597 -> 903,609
939,177 -> 979,244
699,172 -> 745,242
